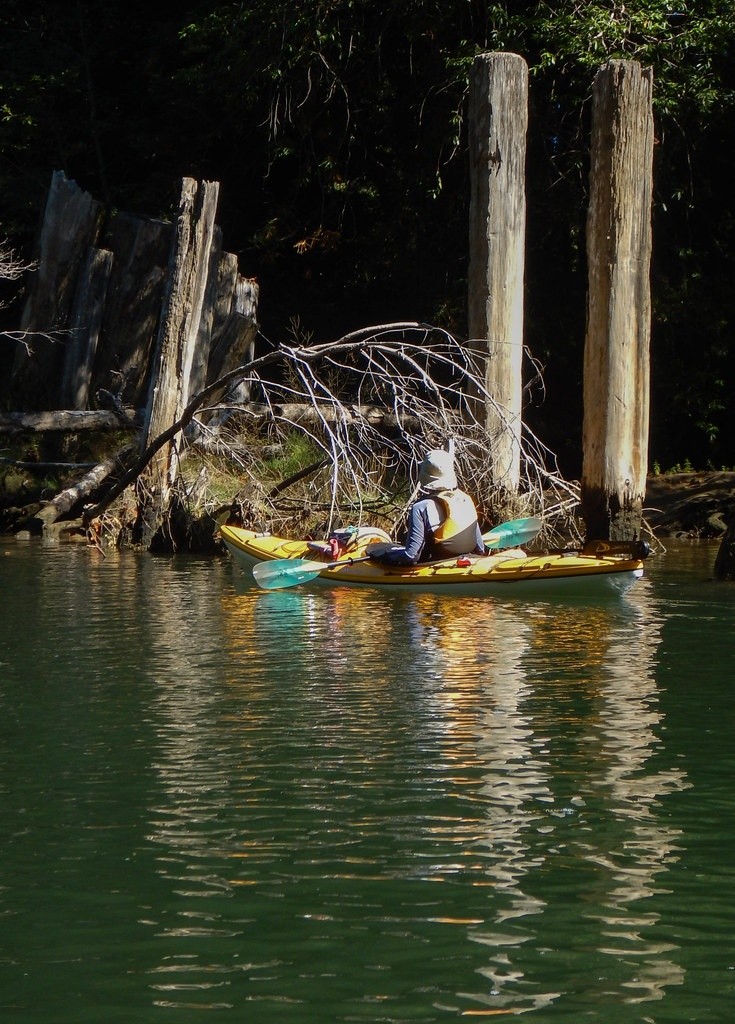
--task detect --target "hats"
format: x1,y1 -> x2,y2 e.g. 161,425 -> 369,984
420,450 -> 458,490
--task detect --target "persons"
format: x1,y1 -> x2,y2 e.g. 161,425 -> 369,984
364,450 -> 485,567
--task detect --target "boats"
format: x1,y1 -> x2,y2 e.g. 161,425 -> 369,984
220,522 -> 651,598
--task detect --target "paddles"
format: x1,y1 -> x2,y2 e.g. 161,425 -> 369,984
250,516 -> 544,591
